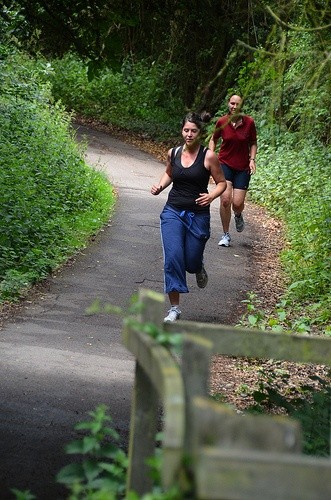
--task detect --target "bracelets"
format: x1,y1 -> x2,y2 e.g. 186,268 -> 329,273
249,158 -> 254,161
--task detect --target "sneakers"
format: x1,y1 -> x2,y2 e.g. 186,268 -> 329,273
164,305 -> 180,321
196,266 -> 207,289
234,211 -> 247,234
217,232 -> 231,248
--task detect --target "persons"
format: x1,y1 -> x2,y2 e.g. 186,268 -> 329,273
151,113 -> 227,322
209,94 -> 257,247
